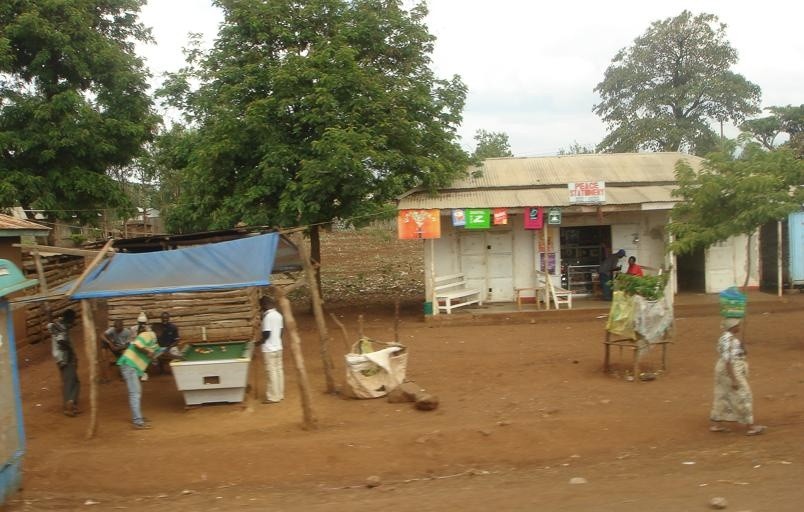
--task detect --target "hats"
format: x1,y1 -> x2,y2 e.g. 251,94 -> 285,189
138,313 -> 149,322
257,296 -> 272,311
618,249 -> 626,257
724,318 -> 742,332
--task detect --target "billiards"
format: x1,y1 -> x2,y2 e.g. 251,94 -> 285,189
220,346 -> 226,352
194,346 -> 214,354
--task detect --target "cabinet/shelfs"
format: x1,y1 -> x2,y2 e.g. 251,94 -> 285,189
567,265 -> 601,298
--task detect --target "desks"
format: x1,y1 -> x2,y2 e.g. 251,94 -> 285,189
169,340 -> 256,407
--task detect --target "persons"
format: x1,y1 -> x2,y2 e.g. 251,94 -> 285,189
154,311 -> 179,377
114,322 -> 186,428
46,309 -> 83,416
598,247 -> 627,302
625,256 -> 643,277
707,318 -> 768,436
130,311 -> 155,381
99,319 -> 131,382
251,295 -> 284,404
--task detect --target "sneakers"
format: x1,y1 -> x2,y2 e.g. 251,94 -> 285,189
748,425 -> 768,435
65,405 -> 83,415
708,425 -> 732,432
130,416 -> 152,429
260,397 -> 287,403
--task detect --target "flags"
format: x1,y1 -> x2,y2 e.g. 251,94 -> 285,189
463,207 -> 492,229
492,207 -> 509,226
451,208 -> 466,226
522,206 -> 545,230
397,209 -> 441,240
547,206 -> 563,226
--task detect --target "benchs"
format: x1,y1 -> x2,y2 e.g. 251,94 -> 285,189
433,273 -> 481,315
538,271 -> 572,310
